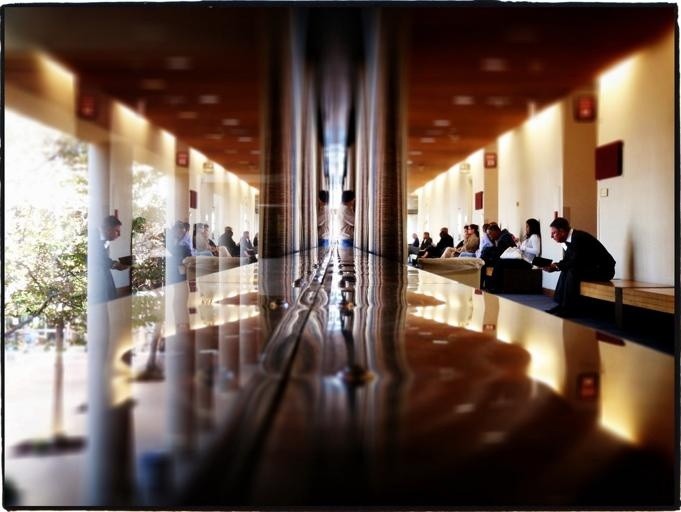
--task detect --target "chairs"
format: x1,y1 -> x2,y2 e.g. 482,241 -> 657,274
415,256 -> 486,291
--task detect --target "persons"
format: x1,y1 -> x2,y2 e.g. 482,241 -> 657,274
318,188 -> 331,247
169,220 -> 258,263
339,188 -> 356,248
97,214 -> 132,300
407,217 -> 616,319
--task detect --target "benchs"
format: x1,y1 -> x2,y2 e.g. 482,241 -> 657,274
579,278 -> 677,329
487,265 -> 543,294
